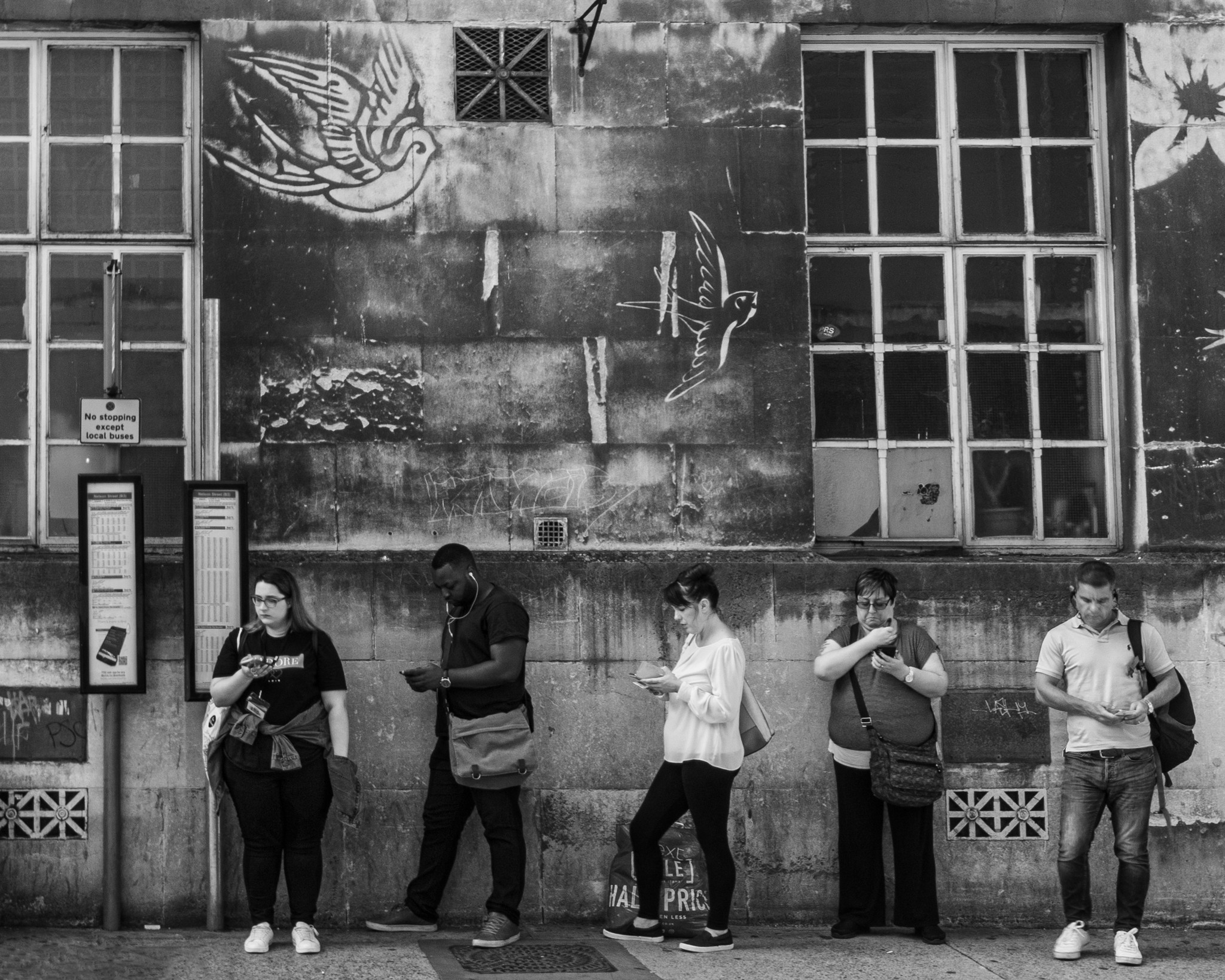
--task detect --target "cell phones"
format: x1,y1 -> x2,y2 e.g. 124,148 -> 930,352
400,671 -> 414,677
875,645 -> 897,663
628,673 -> 640,680
1113,710 -> 1134,716
239,658 -> 273,668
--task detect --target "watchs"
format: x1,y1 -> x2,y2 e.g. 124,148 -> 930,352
899,663 -> 914,683
440,668 -> 451,690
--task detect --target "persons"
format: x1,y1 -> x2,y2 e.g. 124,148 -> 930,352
209,567 -> 348,953
361,542 -> 534,947
602,562 -> 747,951
1035,561 -> 1181,967
813,566 -> 950,945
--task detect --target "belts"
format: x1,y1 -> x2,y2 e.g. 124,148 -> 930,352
1089,747 -> 1138,758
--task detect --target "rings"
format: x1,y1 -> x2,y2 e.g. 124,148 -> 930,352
250,668 -> 257,675
879,665 -> 883,668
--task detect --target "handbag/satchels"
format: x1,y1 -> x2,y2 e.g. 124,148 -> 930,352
1127,618 -> 1197,789
448,705 -> 538,791
201,626 -> 243,756
866,727 -> 946,805
739,678 -> 776,756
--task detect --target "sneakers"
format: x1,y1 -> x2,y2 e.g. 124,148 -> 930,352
603,918 -> 664,942
366,902 -> 437,932
679,929 -> 734,952
1113,927 -> 1143,966
471,912 -> 521,948
244,922 -> 274,953
1053,920 -> 1091,960
291,921 -> 320,952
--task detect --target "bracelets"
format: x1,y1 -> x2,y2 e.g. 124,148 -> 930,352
1141,697 -> 1154,714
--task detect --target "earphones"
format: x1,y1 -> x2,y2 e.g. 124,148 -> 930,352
1070,591 -> 1075,599
1113,589 -> 1118,600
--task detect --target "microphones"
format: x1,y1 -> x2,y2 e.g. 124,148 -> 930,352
468,572 -> 476,580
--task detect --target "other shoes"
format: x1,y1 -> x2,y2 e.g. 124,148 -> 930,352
831,921 -> 869,939
915,925 -> 946,944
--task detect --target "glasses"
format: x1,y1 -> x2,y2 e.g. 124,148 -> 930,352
855,597 -> 891,610
250,596 -> 288,608
